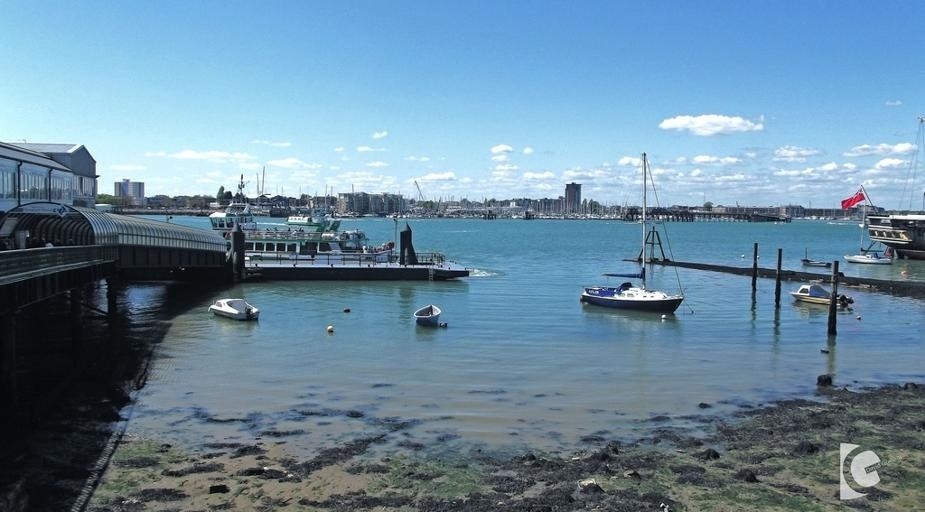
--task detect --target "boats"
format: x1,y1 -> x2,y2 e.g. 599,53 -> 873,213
287,210 -> 341,225
790,282 -> 855,311
207,296 -> 257,321
414,305 -> 443,327
843,252 -> 893,265
233,225 -> 397,263
802,258 -> 833,268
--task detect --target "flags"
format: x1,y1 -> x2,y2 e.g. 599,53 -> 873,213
841,187 -> 867,209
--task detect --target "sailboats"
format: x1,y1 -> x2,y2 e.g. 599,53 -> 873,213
868,113 -> 925,260
584,151 -> 683,312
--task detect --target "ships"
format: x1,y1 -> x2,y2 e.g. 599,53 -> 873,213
208,200 -> 258,232
